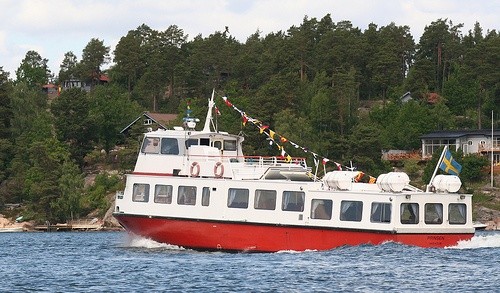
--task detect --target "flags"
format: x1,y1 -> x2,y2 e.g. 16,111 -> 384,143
439,148 -> 462,176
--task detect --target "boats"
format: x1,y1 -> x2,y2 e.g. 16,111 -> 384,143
111,89 -> 476,254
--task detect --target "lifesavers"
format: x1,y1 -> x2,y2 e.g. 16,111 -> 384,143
214,161 -> 225,179
190,161 -> 201,177
